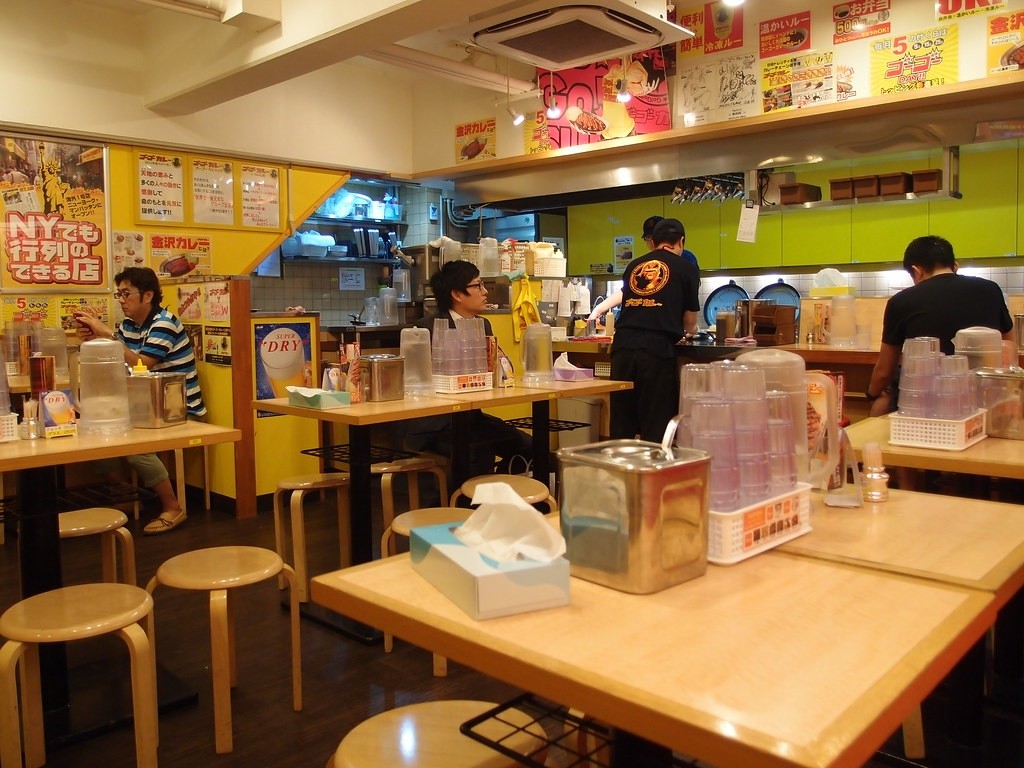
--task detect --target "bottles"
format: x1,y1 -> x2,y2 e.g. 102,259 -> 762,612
861,465 -> 889,501
606,308 -> 614,336
794,331 -> 813,344
132,358 -> 147,375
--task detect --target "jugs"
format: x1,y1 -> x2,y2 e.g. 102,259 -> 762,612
36,329 -> 70,384
822,295 -> 856,349
735,348 -> 837,512
400,326 -> 435,398
364,269 -> 412,326
67,338 -> 131,440
951,327 -> 1004,368
520,322 -> 554,387
438,236 -> 499,276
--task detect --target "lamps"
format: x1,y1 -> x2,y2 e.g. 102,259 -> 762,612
617,56 -> 631,102
547,71 -> 560,118
506,58 -> 525,126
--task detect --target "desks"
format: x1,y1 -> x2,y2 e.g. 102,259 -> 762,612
0,363 -> 1024,768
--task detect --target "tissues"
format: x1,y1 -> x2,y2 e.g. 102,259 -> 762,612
410,480 -> 574,619
553,351 -> 593,381
285,385 -> 351,409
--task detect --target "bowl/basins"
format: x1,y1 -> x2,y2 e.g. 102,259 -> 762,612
763,94 -> 773,100
783,28 -> 808,50
764,103 -> 774,111
331,246 -> 348,256
1000,39 -> 1024,70
834,5 -> 850,19
159,252 -> 199,277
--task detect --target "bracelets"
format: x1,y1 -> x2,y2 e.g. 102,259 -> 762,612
865,387 -> 874,400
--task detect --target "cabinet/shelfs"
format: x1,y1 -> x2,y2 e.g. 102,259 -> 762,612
280,180 -> 408,265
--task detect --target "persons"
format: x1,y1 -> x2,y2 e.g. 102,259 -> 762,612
406,258 -> 532,510
869,366 -> 919,493
864,235 -> 1017,400
587,215 -> 702,444
71,266 -> 209,535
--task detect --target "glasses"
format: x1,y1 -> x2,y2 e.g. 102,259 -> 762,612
114,291 -> 141,299
643,234 -> 652,241
466,280 -> 485,290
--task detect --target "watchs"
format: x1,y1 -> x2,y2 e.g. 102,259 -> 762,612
111,331 -> 119,339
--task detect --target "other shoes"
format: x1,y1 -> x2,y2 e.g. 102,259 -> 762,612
144,509 -> 188,534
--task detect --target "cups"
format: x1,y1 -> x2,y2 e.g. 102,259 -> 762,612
897,336 -> 977,420
1014,314 -> 1024,354
677,360 -> 797,511
0,319 -> 45,416
259,346 -> 312,398
715,312 -> 736,343
432,318 -> 487,375
19,418 -> 40,439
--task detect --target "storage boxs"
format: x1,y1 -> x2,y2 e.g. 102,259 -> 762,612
888,407 -> 987,452
830,178 -> 854,200
912,169 -> 943,192
439,242 -> 567,277
707,483 -> 814,567
853,175 -> 880,199
0,413 -> 20,444
486,336 -> 499,388
806,371 -> 846,488
778,183 -> 822,205
432,372 -> 493,394
879,172 -> 913,196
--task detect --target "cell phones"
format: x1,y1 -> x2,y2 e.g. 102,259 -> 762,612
73,313 -> 94,336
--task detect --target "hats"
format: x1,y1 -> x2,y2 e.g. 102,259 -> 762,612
641,216 -> 664,239
653,218 -> 685,236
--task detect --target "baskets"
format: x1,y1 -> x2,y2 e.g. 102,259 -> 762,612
889,407 -> 988,452
706,480 -> 813,565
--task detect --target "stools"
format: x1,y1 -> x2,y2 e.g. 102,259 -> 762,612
0,582 -> 158,768
142,545 -> 303,754
379,507 -> 476,676
369,457 -> 447,557
326,700 -> 547,768
450,475 -> 557,514
58,507 -> 140,626
273,472 -> 351,604
